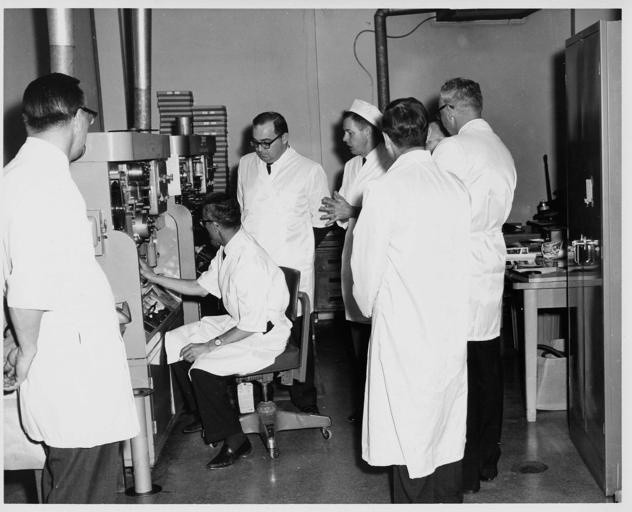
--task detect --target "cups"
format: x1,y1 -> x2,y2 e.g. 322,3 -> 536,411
541,239 -> 562,259
175,116 -> 194,136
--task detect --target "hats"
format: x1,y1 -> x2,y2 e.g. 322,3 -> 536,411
349,99 -> 384,132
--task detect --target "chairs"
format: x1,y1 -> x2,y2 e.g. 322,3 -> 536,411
201,266 -> 333,460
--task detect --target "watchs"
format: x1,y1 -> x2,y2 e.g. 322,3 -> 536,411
214,337 -> 221,346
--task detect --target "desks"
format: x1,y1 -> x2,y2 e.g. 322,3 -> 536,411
502,227 -> 567,424
568,229 -> 603,430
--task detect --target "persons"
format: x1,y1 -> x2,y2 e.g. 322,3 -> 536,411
319,99 -> 385,363
426,118 -> 447,152
236,112 -> 332,415
350,96 -> 469,503
1,73 -> 142,503
430,78 -> 517,495
138,192 -> 293,470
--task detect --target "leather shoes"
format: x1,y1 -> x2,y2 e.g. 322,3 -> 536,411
464,482 -> 480,493
184,422 -> 203,433
348,411 -> 362,421
207,438 -> 252,470
480,469 -> 497,480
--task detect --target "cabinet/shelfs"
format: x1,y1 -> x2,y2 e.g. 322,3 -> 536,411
314,238 -> 350,311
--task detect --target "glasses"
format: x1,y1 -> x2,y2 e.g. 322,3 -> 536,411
73,106 -> 98,126
250,134 -> 282,149
435,104 -> 454,120
199,220 -> 219,227
